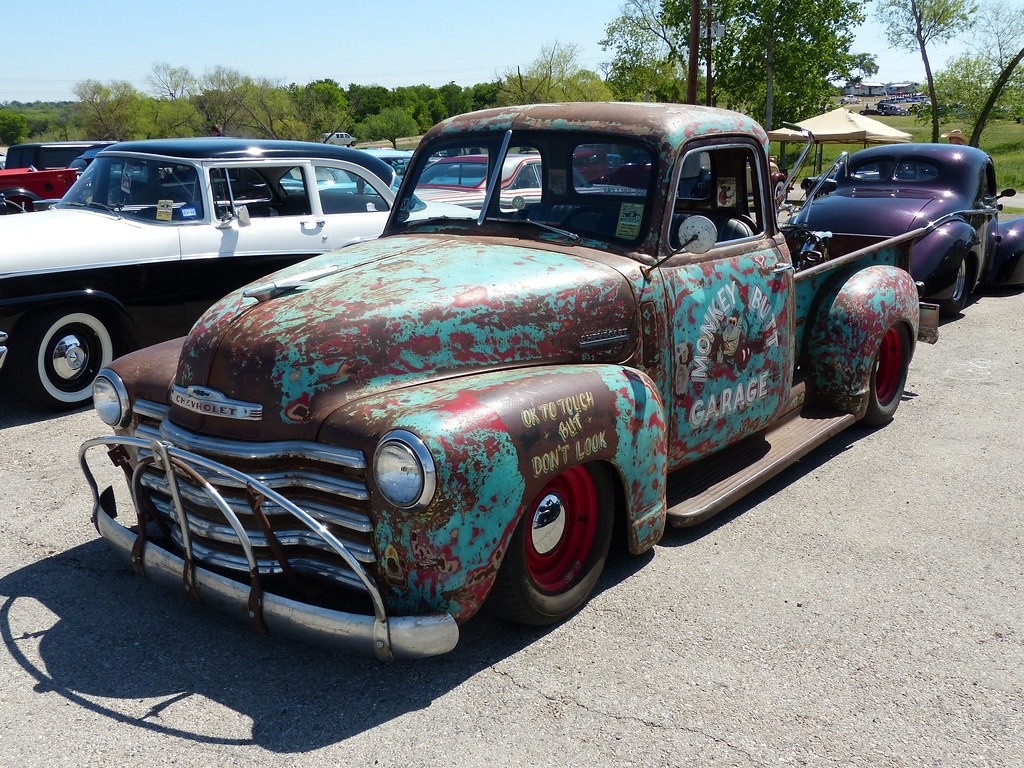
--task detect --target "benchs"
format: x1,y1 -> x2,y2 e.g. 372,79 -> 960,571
528,203 -> 712,245
270,191 -> 382,217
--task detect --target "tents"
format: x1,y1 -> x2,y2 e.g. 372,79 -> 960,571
768,106 -> 914,185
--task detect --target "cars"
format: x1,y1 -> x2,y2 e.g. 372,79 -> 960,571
0,100 -> 939,670
841,93 -> 929,117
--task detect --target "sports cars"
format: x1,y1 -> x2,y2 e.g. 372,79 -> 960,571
781,140 -> 1024,315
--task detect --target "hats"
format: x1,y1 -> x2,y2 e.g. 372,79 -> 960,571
941,130 -> 968,145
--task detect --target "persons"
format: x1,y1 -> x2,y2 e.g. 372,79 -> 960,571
941,128 -> 969,145
211,124 -> 226,137
